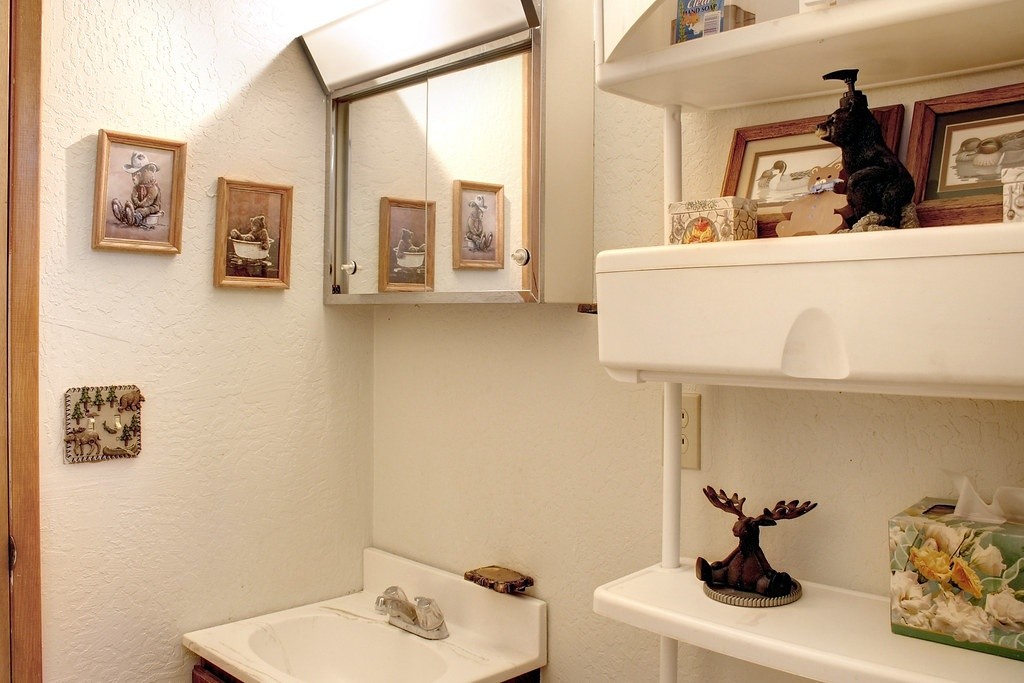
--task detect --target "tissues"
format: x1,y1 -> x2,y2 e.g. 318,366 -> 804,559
887,474 -> 1024,663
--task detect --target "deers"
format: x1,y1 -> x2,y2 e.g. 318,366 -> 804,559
698,486 -> 817,598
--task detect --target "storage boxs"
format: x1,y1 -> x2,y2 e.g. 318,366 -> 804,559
666,195 -> 760,246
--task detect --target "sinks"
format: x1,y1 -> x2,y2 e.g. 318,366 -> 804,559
181,545 -> 549,683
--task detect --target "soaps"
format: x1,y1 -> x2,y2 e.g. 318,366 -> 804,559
463,565 -> 534,594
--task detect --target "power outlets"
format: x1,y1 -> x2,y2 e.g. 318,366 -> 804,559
658,392 -> 701,469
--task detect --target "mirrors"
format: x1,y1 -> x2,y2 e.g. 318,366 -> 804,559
326,25 -> 539,308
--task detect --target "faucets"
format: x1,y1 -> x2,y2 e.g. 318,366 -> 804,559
373,594 -> 416,628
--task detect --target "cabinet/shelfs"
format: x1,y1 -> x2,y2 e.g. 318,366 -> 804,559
591,0 -> 1024,683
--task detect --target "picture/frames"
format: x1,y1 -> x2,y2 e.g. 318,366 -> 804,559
211,177 -> 295,288
903,80 -> 1024,228
452,179 -> 504,271
721,104 -> 905,239
377,196 -> 437,294
92,128 -> 186,255
